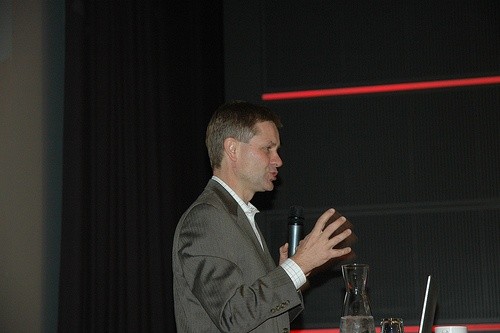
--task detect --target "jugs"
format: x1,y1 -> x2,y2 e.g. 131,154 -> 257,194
340,264 -> 377,333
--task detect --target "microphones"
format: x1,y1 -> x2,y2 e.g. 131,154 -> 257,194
288,205 -> 304,259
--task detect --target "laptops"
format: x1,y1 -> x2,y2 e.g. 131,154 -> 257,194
417,274 -> 440,333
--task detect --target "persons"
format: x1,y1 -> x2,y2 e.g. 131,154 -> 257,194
170,100 -> 353,333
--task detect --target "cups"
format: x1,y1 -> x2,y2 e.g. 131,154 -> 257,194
382,318 -> 404,333
